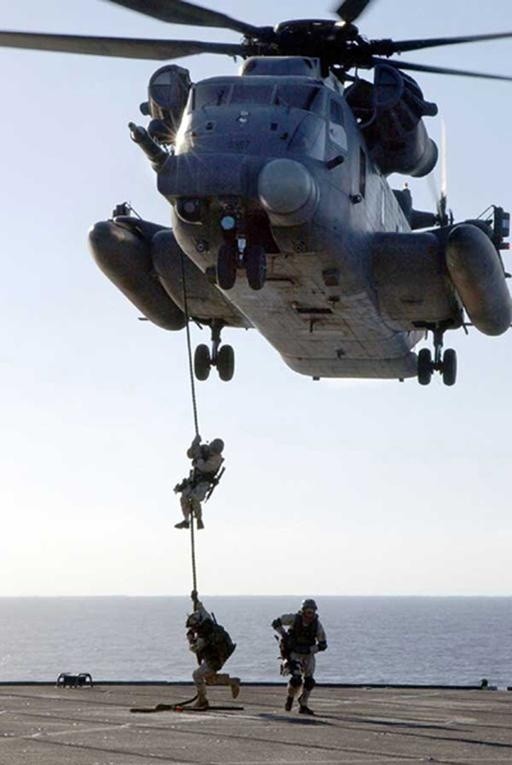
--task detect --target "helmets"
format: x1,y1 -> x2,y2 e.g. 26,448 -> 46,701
211,439 -> 224,453
187,613 -> 202,627
302,599 -> 317,610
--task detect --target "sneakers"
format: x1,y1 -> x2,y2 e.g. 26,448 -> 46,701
174,521 -> 189,528
286,696 -> 294,710
231,678 -> 240,698
197,520 -> 203,528
190,701 -> 208,708
299,705 -> 312,714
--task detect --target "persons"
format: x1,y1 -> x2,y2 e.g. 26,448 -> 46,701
270,598 -> 327,714
183,590 -> 241,708
173,432 -> 227,529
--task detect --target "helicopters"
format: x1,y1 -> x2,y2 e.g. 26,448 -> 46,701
0,0 -> 512,386
